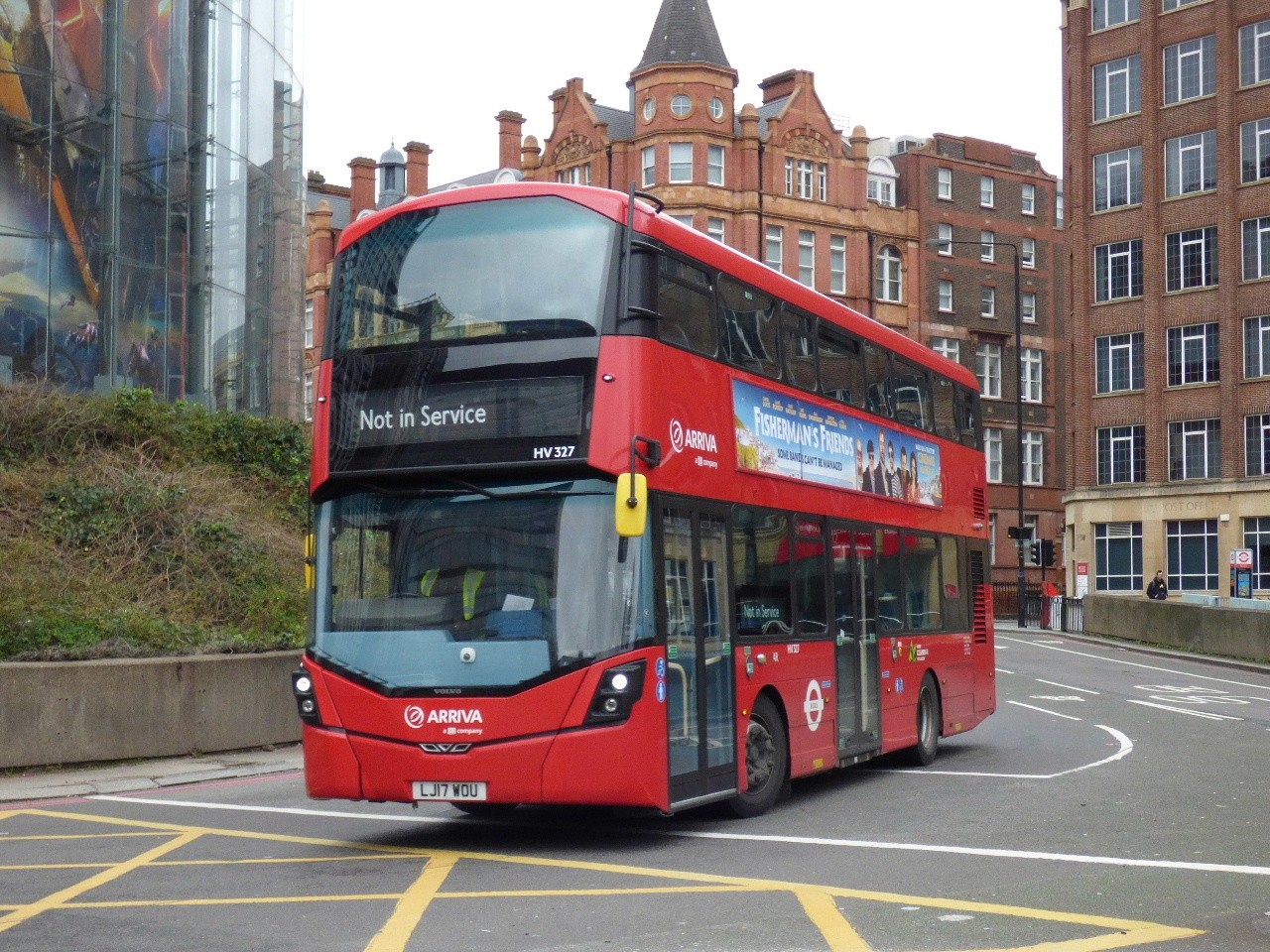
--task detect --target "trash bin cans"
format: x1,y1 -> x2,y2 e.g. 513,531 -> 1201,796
1049,595 -> 1083,631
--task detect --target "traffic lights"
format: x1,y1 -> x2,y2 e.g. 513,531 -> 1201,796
1030,539 -> 1042,566
1008,526 -> 1024,540
1044,540 -> 1056,566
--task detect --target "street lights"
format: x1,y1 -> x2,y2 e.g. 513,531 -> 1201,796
924,238 -> 1027,629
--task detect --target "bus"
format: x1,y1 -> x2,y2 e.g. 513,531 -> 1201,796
292,181 -> 997,818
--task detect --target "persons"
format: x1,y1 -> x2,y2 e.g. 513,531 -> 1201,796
1146,570 -> 1168,600
856,428 -> 922,503
422,538 -> 489,629
